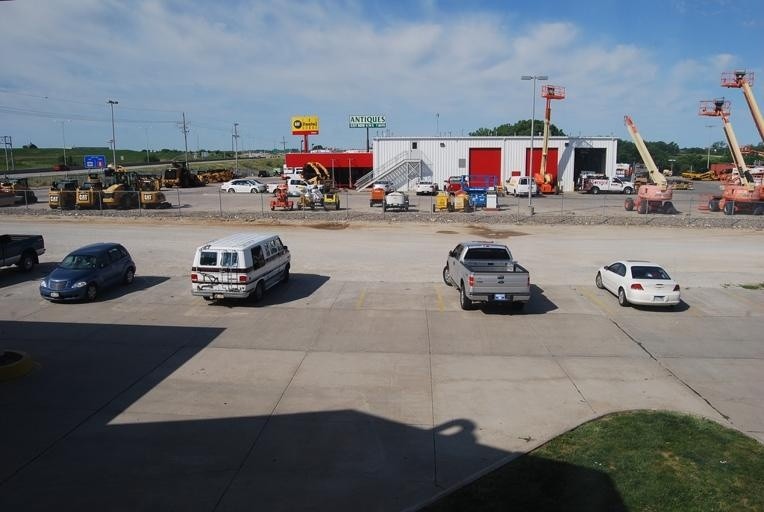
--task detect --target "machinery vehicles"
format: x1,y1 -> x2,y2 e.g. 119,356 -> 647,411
268,161 -> 339,212
534,86 -> 567,195
160,161 -> 239,185
0,170 -> 171,209
617,68 -> 763,215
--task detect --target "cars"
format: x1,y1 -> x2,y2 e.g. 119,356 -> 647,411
107,162 -> 120,168
39,241 -> 135,305
594,259 -> 681,310
220,164 -> 319,197
368,175 -> 499,214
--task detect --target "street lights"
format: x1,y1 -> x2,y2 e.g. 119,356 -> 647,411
233,123 -> 239,177
435,112 -> 440,138
231,135 -> 239,159
62,118 -> 71,165
520,75 -> 548,208
107,97 -> 121,170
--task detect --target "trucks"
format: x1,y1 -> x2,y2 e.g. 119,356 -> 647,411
575,169 -> 636,195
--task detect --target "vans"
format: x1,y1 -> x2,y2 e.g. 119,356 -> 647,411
191,233 -> 292,308
504,175 -> 536,198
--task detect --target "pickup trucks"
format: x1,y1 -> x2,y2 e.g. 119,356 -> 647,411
441,241 -> 531,312
0,231 -> 46,274
